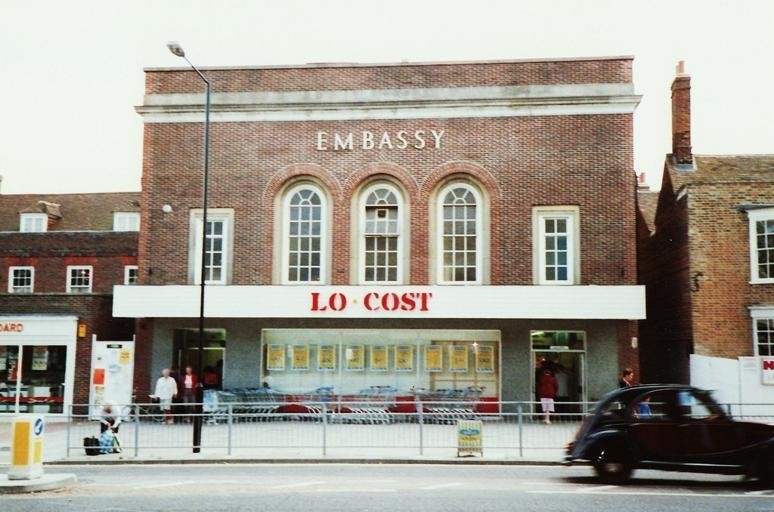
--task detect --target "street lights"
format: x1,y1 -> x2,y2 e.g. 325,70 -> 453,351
164,42 -> 212,454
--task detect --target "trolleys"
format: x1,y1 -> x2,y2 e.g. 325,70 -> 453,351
214,386 -> 484,426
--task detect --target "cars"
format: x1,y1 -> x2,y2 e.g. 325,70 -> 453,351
562,382 -> 774,490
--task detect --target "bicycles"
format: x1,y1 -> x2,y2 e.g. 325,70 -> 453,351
121,388 -> 168,422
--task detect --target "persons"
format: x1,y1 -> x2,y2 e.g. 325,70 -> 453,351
616,367 -> 634,388
536,366 -> 558,424
99,405 -> 123,452
177,365 -> 199,423
555,364 -> 569,420
638,396 -> 652,420
152,367 -> 178,424
202,364 -> 219,389
